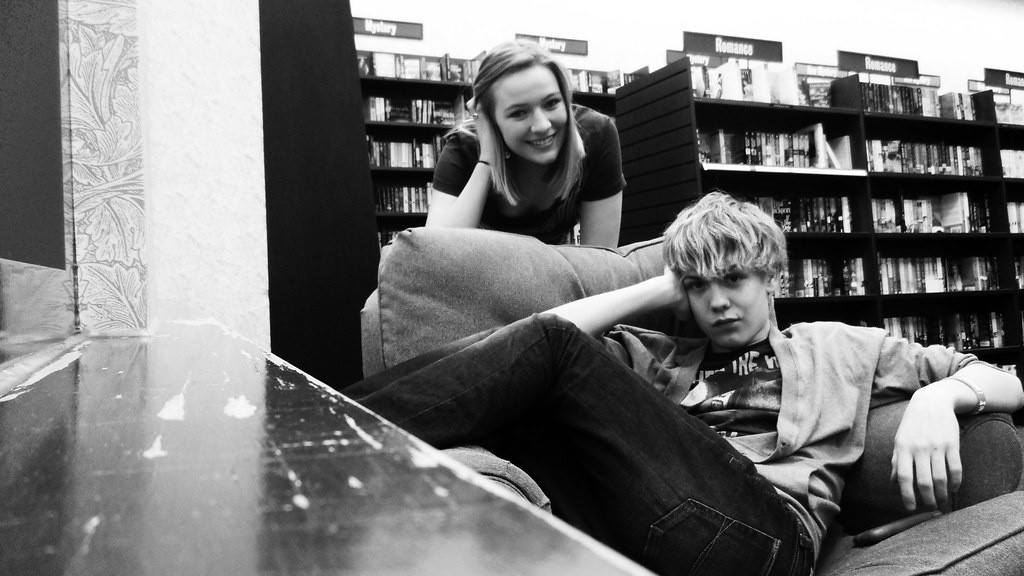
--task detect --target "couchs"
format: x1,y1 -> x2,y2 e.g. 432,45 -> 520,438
359,282 -> 1024,576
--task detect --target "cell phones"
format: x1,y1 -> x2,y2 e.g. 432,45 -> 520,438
852,508 -> 944,549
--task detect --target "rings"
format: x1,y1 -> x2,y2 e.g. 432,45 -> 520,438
472,113 -> 481,120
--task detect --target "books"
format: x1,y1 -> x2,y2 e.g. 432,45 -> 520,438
687,57 -> 1022,355
356,53 -> 648,258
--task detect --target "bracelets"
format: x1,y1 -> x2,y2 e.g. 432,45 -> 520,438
477,159 -> 492,166
950,376 -> 986,417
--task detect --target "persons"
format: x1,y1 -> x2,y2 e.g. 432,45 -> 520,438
339,190 -> 1020,576
425,40 -> 625,247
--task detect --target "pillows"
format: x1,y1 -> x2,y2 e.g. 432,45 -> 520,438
373,226 -> 671,382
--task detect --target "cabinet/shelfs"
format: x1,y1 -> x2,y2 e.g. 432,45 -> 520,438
353,43 -> 1024,416
